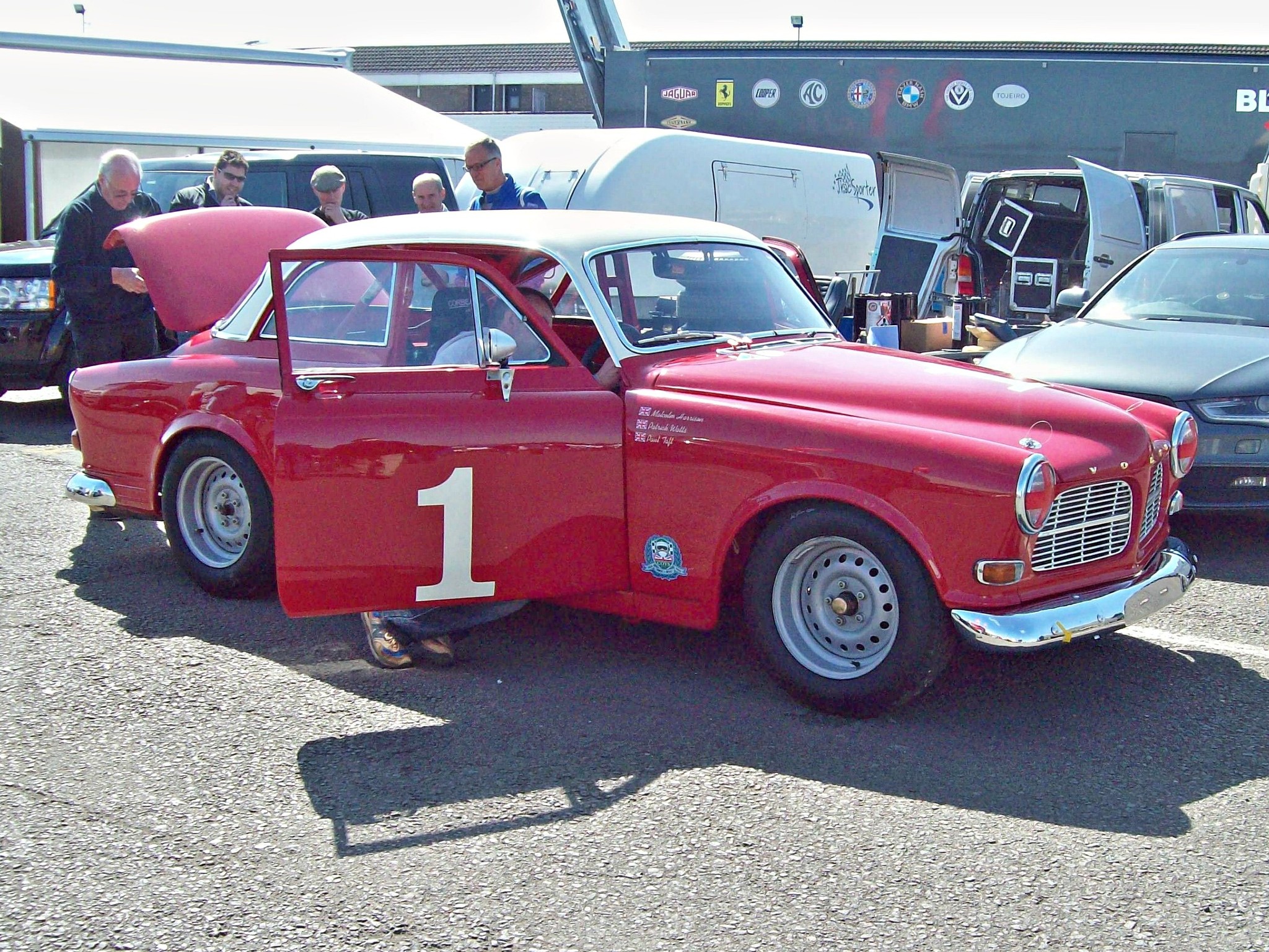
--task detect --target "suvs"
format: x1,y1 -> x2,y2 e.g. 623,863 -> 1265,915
0,144 -> 464,417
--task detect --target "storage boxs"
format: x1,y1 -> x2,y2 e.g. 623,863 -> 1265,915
900,316 -> 954,354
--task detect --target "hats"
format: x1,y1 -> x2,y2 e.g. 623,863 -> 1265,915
310,165 -> 347,193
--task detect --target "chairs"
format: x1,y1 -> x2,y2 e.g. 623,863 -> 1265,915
812,274 -> 848,328
427,286 -> 490,367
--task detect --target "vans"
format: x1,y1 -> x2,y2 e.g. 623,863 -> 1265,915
855,151 -> 1269,353
456,130 -> 882,322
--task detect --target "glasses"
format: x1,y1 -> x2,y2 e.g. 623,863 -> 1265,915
462,157 -> 496,172
218,168 -> 246,182
103,173 -> 144,198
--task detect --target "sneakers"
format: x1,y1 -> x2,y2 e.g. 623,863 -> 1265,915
410,632 -> 456,666
360,611 -> 413,668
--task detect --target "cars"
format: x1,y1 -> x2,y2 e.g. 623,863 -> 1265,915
976,232 -> 1269,522
59,203 -> 1200,716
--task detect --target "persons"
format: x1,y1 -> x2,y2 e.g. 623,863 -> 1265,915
453,138 -> 549,288
402,172 -> 463,311
47,146 -> 166,370
167,149 -> 253,214
357,286 -> 621,670
304,164 -> 370,227
